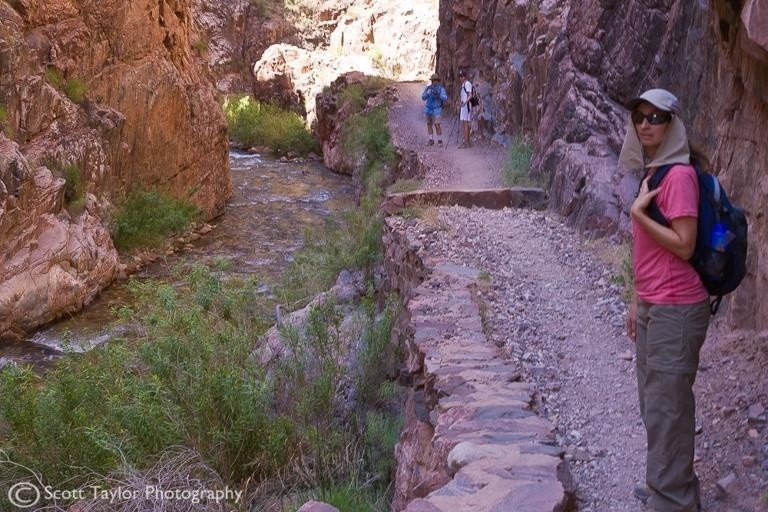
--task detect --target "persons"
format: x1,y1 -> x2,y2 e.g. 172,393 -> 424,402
421,74 -> 448,147
616,87 -> 710,512
456,70 -> 474,148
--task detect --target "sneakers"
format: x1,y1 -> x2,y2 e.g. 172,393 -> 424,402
635,485 -> 657,500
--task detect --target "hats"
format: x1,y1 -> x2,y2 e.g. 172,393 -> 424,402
625,88 -> 679,112
457,71 -> 466,76
429,74 -> 439,80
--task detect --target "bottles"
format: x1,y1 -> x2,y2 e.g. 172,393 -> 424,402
708,223 -> 729,280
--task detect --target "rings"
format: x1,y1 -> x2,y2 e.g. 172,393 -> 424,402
640,188 -> 643,192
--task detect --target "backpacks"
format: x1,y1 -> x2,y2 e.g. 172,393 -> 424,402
636,158 -> 747,315
463,81 -> 479,112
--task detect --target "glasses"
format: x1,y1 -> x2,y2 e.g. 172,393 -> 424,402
632,111 -> 669,124
